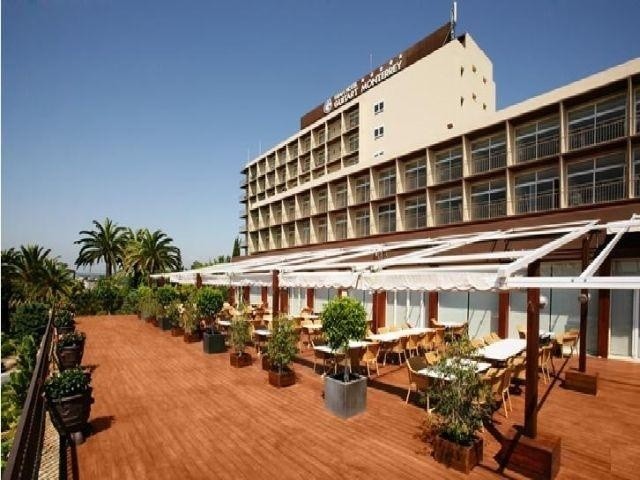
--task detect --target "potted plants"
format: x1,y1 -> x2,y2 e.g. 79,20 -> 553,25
54,309 -> 76,336
41,367 -> 93,446
130,284 -> 369,419
422,330 -> 492,473
56,333 -> 86,371
